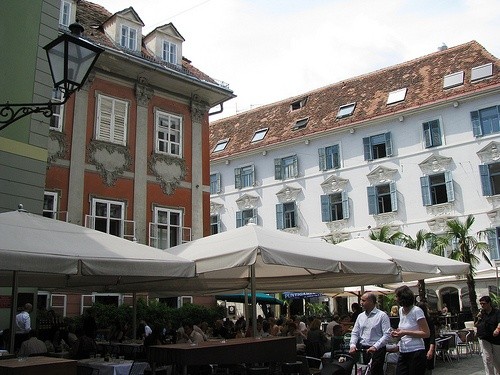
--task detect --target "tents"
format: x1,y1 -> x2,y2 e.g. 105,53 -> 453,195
148,217 -> 469,337
0,203 -> 198,357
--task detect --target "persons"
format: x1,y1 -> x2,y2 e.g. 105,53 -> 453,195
382,302 -> 475,359
136,303 -> 363,375
474,296 -> 500,375
0,303 -> 125,375
417,304 -> 435,375
390,285 -> 431,375
349,292 -> 391,375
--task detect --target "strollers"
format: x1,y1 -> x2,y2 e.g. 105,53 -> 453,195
319,348 -> 374,375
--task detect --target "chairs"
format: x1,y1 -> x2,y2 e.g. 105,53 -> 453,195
77,323 -> 478,375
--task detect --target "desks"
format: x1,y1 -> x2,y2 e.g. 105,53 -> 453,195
0,355 -> 78,375
95,339 -> 145,362
148,334 -> 297,375
77,356 -> 135,375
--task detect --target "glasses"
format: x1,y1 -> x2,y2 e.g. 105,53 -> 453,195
480,303 -> 488,306
